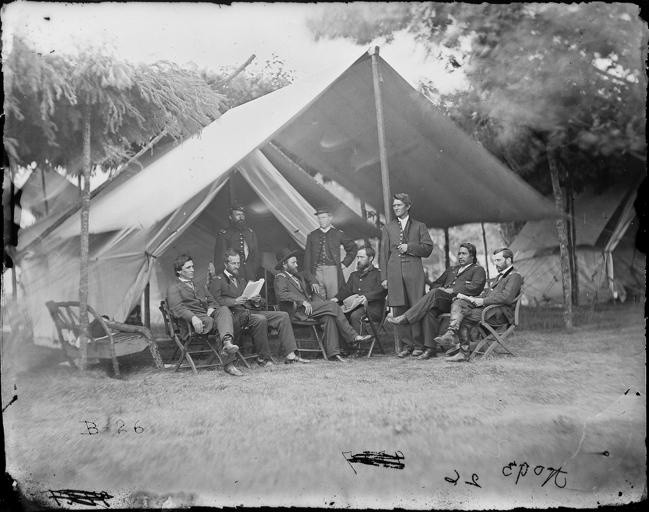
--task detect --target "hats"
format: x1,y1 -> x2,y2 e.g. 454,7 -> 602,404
276,248 -> 297,269
342,294 -> 363,313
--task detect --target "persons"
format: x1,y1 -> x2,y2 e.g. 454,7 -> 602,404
435,248 -> 525,361
330,247 -> 385,360
167,253 -> 244,377
209,248 -> 312,367
388,243 -> 486,358
303,208 -> 358,300
381,193 -> 434,357
213,202 -> 261,284
273,249 -> 374,363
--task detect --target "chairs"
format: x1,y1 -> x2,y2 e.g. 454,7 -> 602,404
46,298 -> 165,377
155,296 -> 518,374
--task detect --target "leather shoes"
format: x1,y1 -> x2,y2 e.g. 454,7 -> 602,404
352,335 -> 370,343
224,366 -> 241,376
285,356 -> 309,363
223,341 -> 239,353
397,348 -> 470,361
388,315 -> 408,325
